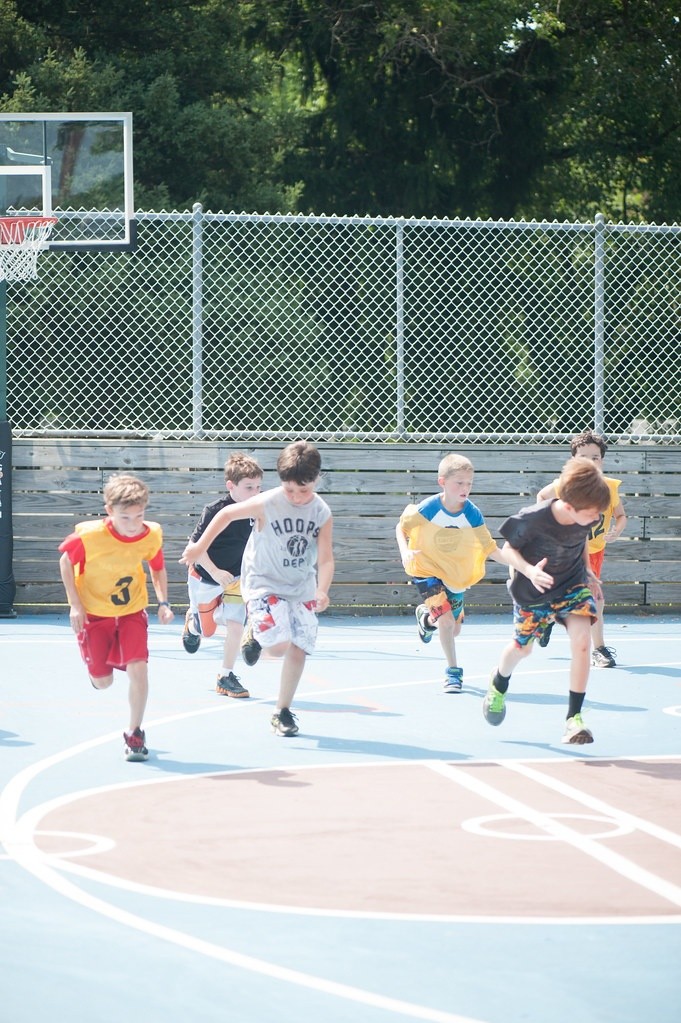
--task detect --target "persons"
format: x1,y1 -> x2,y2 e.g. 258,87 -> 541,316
176,439 -> 334,738
396,453 -> 512,693
182,452 -> 264,698
60,476 -> 175,763
482,456 -> 611,746
535,430 -> 626,669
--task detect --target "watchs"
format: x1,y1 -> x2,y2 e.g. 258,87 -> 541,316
157,601 -> 170,611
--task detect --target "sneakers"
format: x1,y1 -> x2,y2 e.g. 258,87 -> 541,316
269,708 -> 299,737
591,644 -> 616,668
181,607 -> 200,654
123,726 -> 149,761
215,671 -> 249,698
415,604 -> 438,643
563,711 -> 594,745
238,621 -> 262,666
442,664 -> 463,693
481,668 -> 507,726
